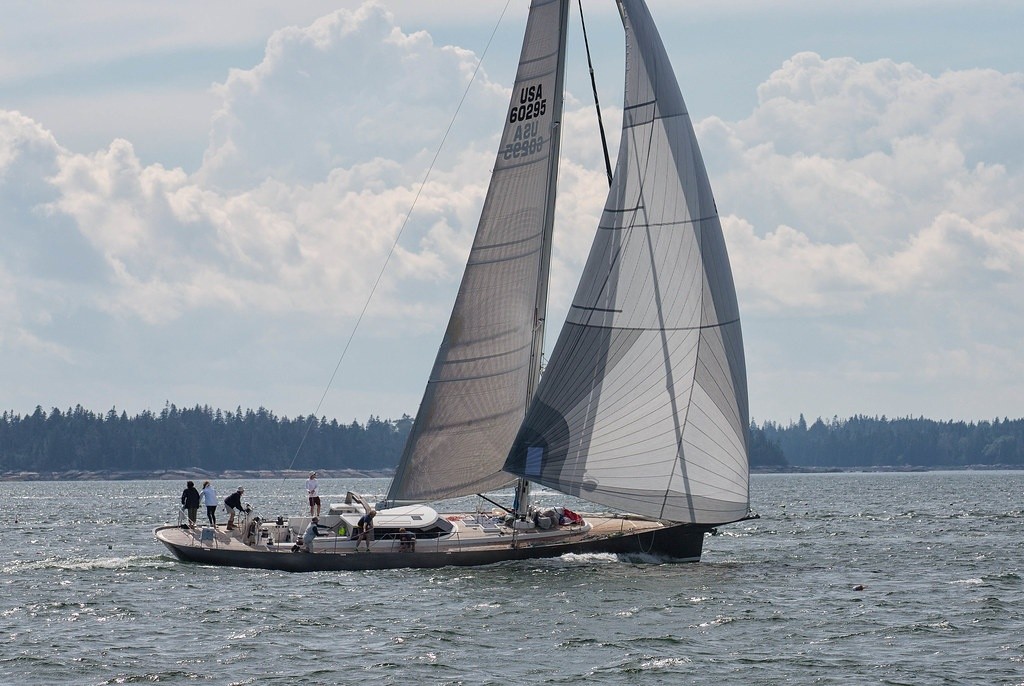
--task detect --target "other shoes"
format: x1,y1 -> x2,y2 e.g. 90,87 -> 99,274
366,547 -> 371,553
210,524 -> 216,527
354,547 -> 359,552
290,545 -> 301,553
226,520 -> 238,531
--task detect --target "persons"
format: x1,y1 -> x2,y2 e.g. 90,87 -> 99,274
199,481 -> 218,529
399,528 -> 416,552
305,471 -> 321,519
303,517 -> 333,553
354,511 -> 377,553
181,481 -> 200,528
247,517 -> 262,546
224,487 -> 246,530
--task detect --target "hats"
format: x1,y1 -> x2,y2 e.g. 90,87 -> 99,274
237,486 -> 245,492
308,471 -> 316,479
311,516 -> 320,525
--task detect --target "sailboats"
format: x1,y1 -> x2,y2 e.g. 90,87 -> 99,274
152,0 -> 760,572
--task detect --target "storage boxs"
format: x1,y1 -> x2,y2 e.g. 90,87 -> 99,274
202,526 -> 214,540
